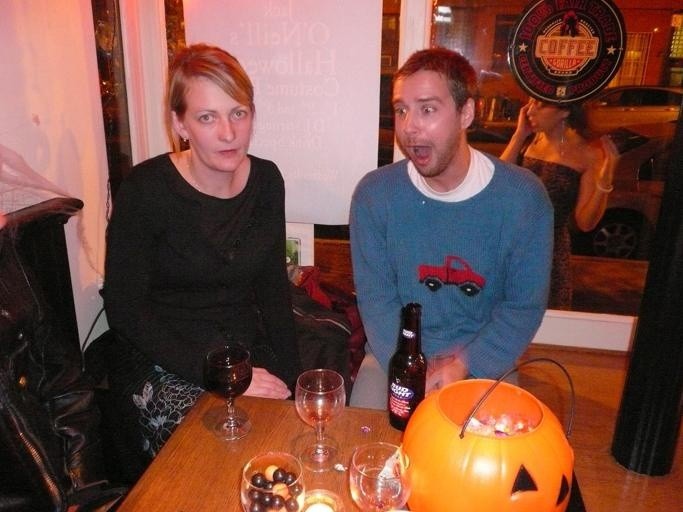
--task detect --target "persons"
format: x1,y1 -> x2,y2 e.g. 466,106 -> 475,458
98,41 -> 352,405
497,94 -> 619,310
347,46 -> 555,412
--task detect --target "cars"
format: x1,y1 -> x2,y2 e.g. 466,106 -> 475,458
580,84 -> 683,150
564,120 -> 676,260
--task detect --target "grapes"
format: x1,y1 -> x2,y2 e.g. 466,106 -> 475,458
247,464 -> 304,512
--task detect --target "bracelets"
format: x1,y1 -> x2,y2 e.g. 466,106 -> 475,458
595,180 -> 615,195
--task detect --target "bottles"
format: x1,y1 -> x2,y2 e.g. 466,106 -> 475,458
387,303 -> 428,433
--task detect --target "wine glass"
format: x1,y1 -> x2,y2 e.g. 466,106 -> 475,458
294,369 -> 347,474
206,346 -> 253,441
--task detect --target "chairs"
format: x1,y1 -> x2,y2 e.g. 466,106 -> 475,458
1,309 -> 129,512
292,303 -> 352,400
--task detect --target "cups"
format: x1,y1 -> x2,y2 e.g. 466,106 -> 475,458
349,441 -> 411,511
240,451 -> 305,511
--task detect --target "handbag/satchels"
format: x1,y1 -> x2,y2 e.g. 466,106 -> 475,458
289,267 -> 365,379
